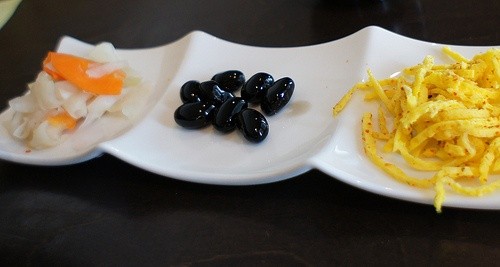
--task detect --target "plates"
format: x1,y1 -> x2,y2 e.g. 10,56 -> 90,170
0,35 -> 500,212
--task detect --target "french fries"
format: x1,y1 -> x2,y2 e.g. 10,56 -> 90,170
333,46 -> 500,214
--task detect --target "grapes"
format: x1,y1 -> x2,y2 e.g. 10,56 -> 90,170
174,70 -> 295,143
